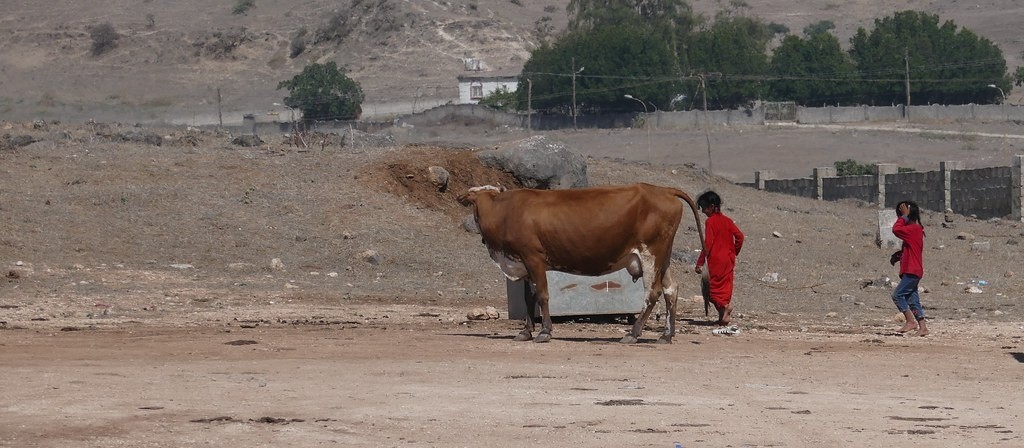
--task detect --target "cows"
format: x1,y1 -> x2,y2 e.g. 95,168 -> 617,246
457,185 -> 712,344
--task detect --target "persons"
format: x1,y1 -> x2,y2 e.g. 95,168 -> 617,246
695,191 -> 744,326
890,201 -> 929,338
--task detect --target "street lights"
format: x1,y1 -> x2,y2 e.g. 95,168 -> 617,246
526,78 -> 532,136
272,102 -> 296,122
624,94 -> 651,165
573,66 -> 585,132
987,84 -> 1007,136
